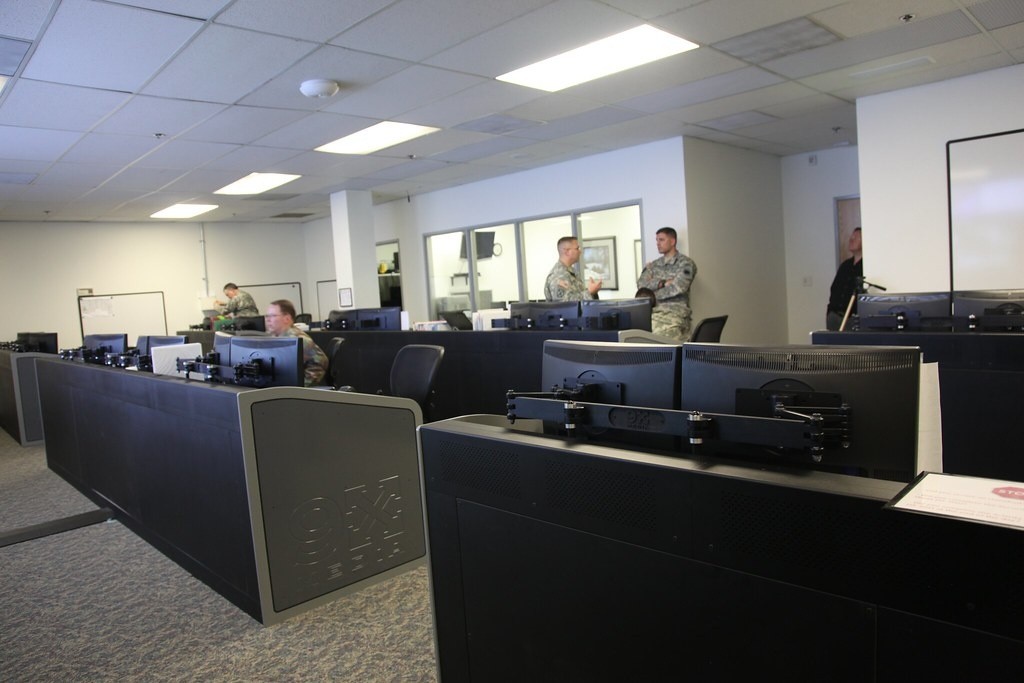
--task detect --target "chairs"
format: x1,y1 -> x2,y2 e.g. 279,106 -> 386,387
377,344 -> 445,414
326,337 -> 346,388
295,313 -> 311,332
690,315 -> 728,342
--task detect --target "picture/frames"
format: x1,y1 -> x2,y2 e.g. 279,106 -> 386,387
571,236 -> 618,290
634,239 -> 642,282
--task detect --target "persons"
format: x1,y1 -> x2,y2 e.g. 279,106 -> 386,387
827,227 -> 863,331
544,237 -> 602,302
265,299 -> 329,387
637,227 -> 698,341
213,282 -> 259,317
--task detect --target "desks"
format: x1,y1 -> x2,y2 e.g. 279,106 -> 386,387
0,342 -> 64,447
178,330 -> 269,358
304,328 -> 682,421
416,415 -> 1024,683
35,358 -> 429,627
810,331 -> 1024,482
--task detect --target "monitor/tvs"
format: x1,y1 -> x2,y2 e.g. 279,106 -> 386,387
2,289 -> 1024,483
460,231 -> 495,259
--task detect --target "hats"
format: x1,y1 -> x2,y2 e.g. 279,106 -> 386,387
636,287 -> 656,308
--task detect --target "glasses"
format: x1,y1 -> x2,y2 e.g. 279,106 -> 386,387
265,313 -> 286,320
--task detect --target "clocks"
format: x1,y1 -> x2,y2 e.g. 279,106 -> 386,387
492,243 -> 502,255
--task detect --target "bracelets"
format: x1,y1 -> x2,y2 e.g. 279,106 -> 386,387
662,280 -> 666,287
